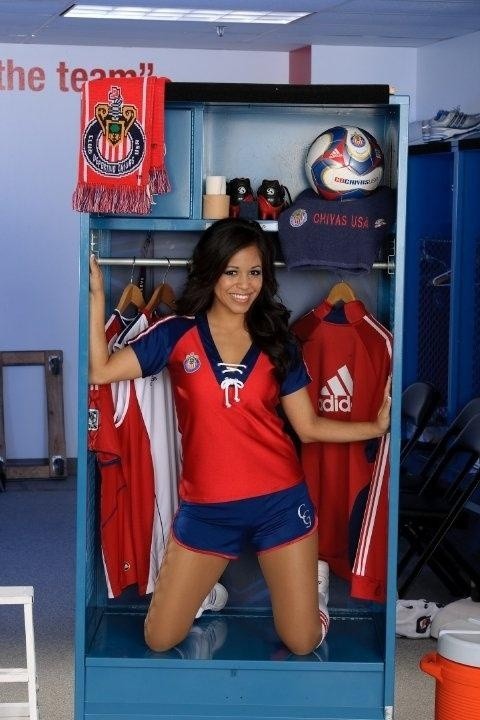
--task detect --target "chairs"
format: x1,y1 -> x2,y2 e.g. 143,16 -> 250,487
399,377 -> 480,608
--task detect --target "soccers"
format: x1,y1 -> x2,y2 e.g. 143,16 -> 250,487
304,125 -> 385,201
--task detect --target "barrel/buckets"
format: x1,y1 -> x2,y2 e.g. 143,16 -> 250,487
419,628 -> 480,720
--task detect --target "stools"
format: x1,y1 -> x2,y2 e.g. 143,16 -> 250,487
0,584 -> 42,719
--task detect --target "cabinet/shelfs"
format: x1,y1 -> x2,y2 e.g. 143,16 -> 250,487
401,123 -> 480,557
69,93 -> 415,720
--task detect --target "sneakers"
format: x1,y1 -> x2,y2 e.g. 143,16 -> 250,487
226,177 -> 257,222
257,180 -> 292,221
421,104 -> 480,143
193,583 -> 229,618
317,560 -> 329,606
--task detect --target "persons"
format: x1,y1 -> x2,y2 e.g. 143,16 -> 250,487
89,216 -> 391,657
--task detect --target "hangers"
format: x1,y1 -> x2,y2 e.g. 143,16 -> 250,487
316,258 -> 359,310
110,255 -> 151,322
432,270 -> 452,288
143,252 -> 183,319
